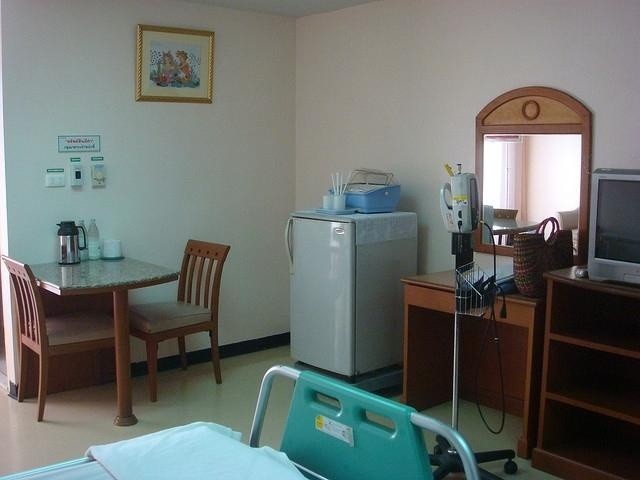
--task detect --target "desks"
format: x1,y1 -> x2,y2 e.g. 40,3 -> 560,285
26,259 -> 179,425
400,272 -> 541,459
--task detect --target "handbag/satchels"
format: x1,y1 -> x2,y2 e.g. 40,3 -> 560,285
513,217 -> 573,298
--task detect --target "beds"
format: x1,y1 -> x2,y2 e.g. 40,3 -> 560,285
0,365 -> 478,479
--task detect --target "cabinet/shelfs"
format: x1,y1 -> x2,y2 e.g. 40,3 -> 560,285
531,267 -> 639,480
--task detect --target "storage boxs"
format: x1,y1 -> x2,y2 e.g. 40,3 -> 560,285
330,183 -> 401,214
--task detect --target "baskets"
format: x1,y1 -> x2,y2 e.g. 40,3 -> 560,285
456,261 -> 497,317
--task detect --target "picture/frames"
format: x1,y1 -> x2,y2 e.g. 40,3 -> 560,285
135,23 -> 215,105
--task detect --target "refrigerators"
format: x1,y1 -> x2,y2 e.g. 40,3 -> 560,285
284,208 -> 419,392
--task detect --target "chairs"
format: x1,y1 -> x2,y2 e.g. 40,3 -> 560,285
1,255 -> 116,422
131,239 -> 230,403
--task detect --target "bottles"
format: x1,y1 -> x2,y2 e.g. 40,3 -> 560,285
76,219 -> 88,261
87,217 -> 101,260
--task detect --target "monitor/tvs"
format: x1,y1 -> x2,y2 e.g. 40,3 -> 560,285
587,168 -> 640,286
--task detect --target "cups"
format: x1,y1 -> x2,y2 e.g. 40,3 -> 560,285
333,195 -> 346,210
103,239 -> 121,257
56,221 -> 86,265
323,195 -> 333,210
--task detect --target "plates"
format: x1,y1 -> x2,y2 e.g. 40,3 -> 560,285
100,255 -> 125,260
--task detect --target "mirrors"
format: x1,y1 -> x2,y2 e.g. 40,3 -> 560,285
474,86 -> 591,264
